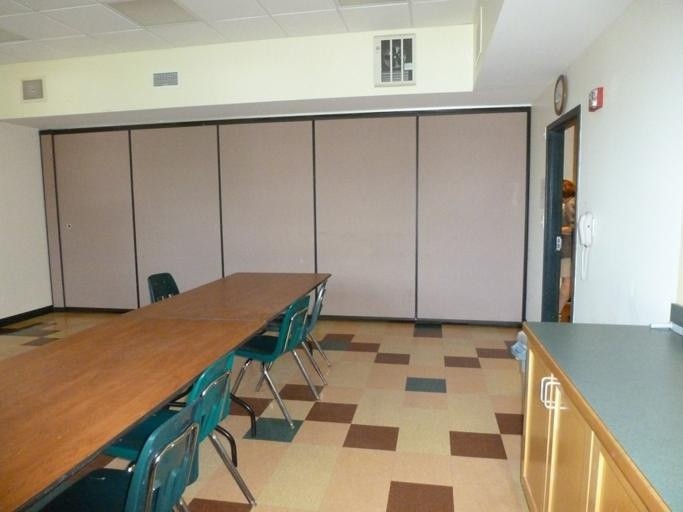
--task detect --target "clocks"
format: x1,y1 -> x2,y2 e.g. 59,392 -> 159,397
553,74 -> 567,114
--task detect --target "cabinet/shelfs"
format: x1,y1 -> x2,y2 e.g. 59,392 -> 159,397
520,341 -> 594,512
594,431 -> 670,512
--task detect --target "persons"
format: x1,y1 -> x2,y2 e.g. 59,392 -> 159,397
558,180 -> 575,321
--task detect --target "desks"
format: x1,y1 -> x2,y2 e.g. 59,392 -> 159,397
0,272 -> 333,512
